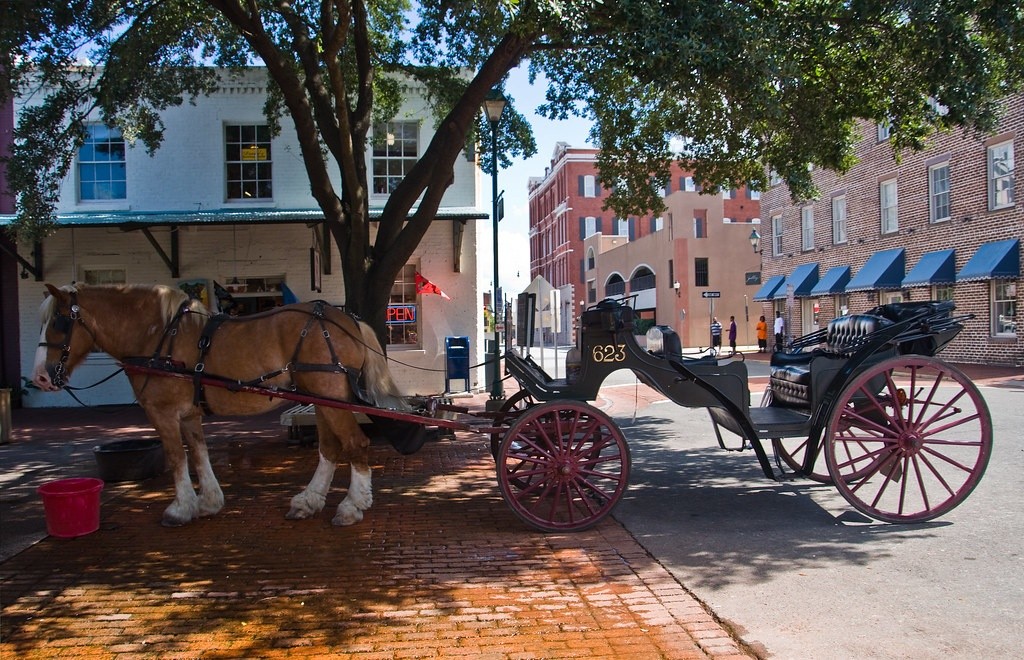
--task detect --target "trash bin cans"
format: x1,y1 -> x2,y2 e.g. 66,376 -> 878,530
445,337 -> 469,379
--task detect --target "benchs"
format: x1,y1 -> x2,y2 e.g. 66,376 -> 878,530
769,314 -> 895,408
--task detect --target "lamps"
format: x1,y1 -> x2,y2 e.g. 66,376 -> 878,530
579,297 -> 585,306
674,280 -> 681,293
748,228 -> 763,255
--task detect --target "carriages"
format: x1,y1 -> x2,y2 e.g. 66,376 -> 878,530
28,274 -> 995,536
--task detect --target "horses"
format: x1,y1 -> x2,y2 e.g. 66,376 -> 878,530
31,278 -> 416,527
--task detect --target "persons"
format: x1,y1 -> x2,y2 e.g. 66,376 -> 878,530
710,316 -> 722,358
725,316 -> 736,355
774,311 -> 784,352
756,315 -> 767,353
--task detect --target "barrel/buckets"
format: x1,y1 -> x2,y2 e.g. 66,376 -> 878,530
34,478 -> 104,537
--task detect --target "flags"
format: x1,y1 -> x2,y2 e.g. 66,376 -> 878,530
415,271 -> 451,302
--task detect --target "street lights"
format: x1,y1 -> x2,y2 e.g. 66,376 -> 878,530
579,299 -> 585,311
673,280 -> 681,295
481,90 -> 519,418
748,229 -> 761,253
